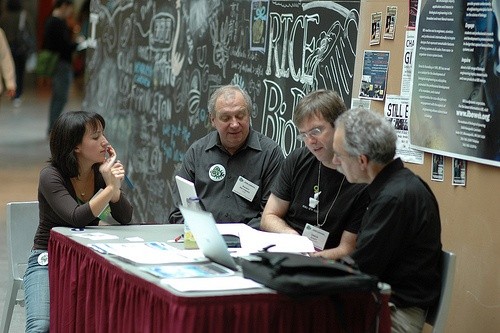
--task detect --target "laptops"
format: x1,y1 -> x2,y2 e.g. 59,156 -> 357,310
174,175 -> 262,272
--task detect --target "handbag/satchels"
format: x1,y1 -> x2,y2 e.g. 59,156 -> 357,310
240,250 -> 380,297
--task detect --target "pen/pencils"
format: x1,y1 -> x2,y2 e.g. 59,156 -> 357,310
174,234 -> 184,242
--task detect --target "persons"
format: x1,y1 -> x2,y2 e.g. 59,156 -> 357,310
0,0 -> 37,107
170,85 -> 284,228
22,111 -> 133,333
260,90 -> 372,256
311,107 -> 441,333
44,0 -> 82,137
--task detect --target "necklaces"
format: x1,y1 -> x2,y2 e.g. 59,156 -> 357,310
77,186 -> 85,196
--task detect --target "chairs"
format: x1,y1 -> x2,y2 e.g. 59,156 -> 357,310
0,201 -> 39,333
422,247 -> 458,333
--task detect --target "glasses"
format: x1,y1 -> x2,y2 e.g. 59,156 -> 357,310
296,122 -> 330,142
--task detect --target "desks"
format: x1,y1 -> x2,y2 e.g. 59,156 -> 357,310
47,224 -> 393,333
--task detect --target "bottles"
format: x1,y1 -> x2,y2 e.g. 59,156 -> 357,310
184,199 -> 198,249
